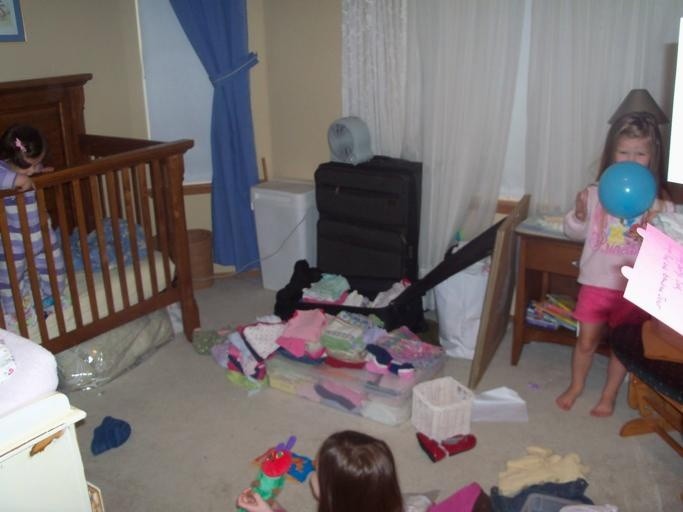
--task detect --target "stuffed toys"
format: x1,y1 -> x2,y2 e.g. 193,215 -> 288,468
238,433 -> 299,512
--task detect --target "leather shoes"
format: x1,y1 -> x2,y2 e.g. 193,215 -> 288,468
417,432 -> 444,462
442,435 -> 476,457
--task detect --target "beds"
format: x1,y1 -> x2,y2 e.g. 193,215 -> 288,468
1,75 -> 200,356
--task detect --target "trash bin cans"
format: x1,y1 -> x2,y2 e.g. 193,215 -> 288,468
184,230 -> 214,288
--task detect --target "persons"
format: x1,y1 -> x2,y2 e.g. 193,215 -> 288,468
0,122 -> 65,321
236,430 -> 434,512
555,110 -> 675,418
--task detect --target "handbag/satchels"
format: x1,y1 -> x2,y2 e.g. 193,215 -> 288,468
434,244 -> 490,360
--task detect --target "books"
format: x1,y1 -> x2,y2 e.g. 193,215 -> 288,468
526,292 -> 576,332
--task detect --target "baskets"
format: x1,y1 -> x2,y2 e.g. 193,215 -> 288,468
412,377 -> 474,439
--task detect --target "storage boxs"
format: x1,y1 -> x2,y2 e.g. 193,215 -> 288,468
268,340 -> 449,426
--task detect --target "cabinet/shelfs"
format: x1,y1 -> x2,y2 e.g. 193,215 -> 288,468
511,214 -> 683,365
315,156 -> 423,331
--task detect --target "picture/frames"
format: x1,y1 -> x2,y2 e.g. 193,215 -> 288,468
0,0 -> 25,44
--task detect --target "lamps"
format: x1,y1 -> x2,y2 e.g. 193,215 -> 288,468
607,88 -> 670,129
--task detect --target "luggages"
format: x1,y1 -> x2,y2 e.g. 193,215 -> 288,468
275,215 -> 506,332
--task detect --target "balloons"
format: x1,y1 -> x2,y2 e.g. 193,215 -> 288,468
598,160 -> 658,220
